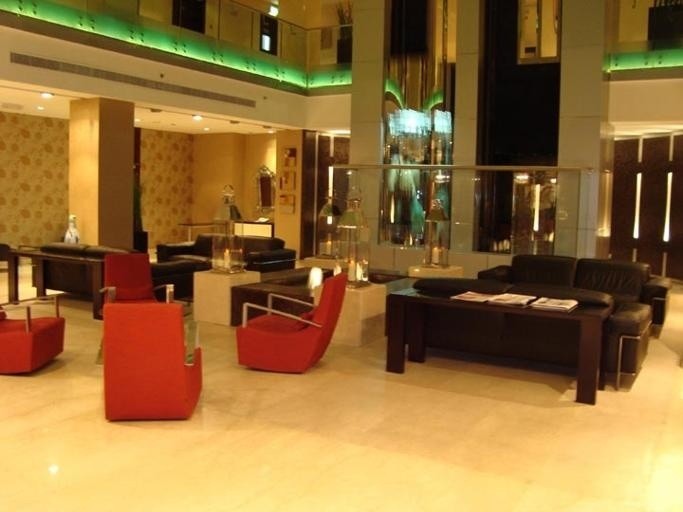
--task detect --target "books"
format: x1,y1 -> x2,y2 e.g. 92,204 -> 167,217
450,291 -> 578,313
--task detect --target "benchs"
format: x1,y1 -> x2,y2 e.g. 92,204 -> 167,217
231,266 -> 332,324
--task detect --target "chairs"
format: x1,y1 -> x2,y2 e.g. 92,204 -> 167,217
96,252 -> 203,422
0,292 -> 66,374
236,273 -> 347,374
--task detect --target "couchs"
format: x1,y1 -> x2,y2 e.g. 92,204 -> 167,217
32,232 -> 296,319
412,252 -> 672,383
